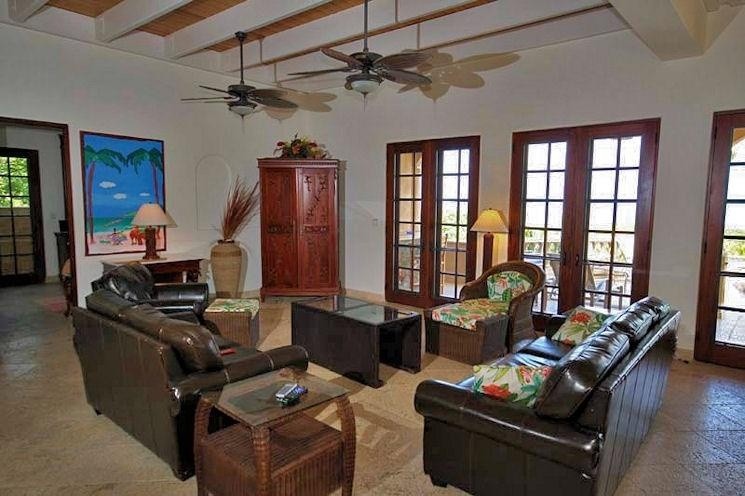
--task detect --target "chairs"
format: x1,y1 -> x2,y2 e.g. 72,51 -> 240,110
91,264 -> 260,351
549,254 -> 628,310
460,261 -> 545,354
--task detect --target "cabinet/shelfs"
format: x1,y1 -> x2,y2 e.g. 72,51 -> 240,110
257,157 -> 342,303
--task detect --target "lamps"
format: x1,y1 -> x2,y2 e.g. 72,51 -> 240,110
130,201 -> 178,260
346,74 -> 382,98
470,208 -> 510,275
227,101 -> 257,119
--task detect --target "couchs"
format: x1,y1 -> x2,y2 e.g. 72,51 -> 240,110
70,288 -> 309,481
414,295 -> 681,496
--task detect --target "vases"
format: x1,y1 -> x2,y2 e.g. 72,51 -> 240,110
209,240 -> 242,298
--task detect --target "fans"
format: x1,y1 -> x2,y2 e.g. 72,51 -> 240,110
180,31 -> 298,108
287,0 -> 433,85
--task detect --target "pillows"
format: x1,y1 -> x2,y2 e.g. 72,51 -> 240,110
472,365 -> 553,409
551,305 -> 613,345
487,271 -> 534,303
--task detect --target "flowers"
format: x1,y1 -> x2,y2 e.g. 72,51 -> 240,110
273,133 -> 328,159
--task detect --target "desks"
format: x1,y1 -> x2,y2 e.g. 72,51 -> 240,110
101,254 -> 204,283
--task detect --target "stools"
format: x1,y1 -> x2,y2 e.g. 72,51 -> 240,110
423,301 -> 509,367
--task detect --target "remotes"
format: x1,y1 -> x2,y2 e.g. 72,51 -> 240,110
275,382 -> 297,398
283,385 -> 308,405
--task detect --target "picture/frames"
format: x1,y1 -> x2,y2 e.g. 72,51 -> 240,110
79,130 -> 166,256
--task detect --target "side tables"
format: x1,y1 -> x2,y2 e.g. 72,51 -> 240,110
194,366 -> 356,496
